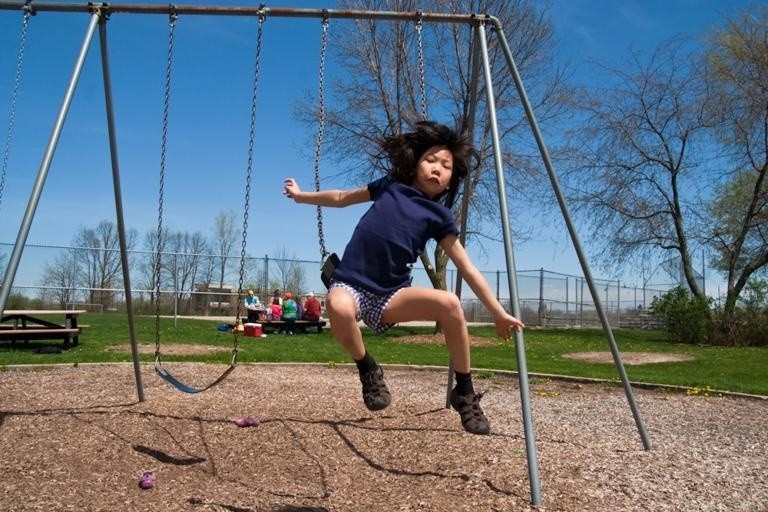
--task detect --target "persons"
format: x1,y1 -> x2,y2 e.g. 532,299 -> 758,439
244,289 -> 322,335
283,121 -> 526,435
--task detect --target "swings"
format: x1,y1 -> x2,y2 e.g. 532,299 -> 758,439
153,9 -> 266,394
314,15 -> 430,289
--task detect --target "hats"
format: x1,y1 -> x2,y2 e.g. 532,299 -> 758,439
286,292 -> 292,298
304,292 -> 314,296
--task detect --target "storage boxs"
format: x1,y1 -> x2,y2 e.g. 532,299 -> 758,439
243,322 -> 262,337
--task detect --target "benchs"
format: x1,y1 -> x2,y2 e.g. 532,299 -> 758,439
242,315 -> 326,334
0,323 -> 91,351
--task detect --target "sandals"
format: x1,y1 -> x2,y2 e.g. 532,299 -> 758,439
451,386 -> 489,435
360,367 -> 391,410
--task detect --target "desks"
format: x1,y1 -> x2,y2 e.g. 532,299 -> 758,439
0,309 -> 87,351
247,306 -> 267,314
